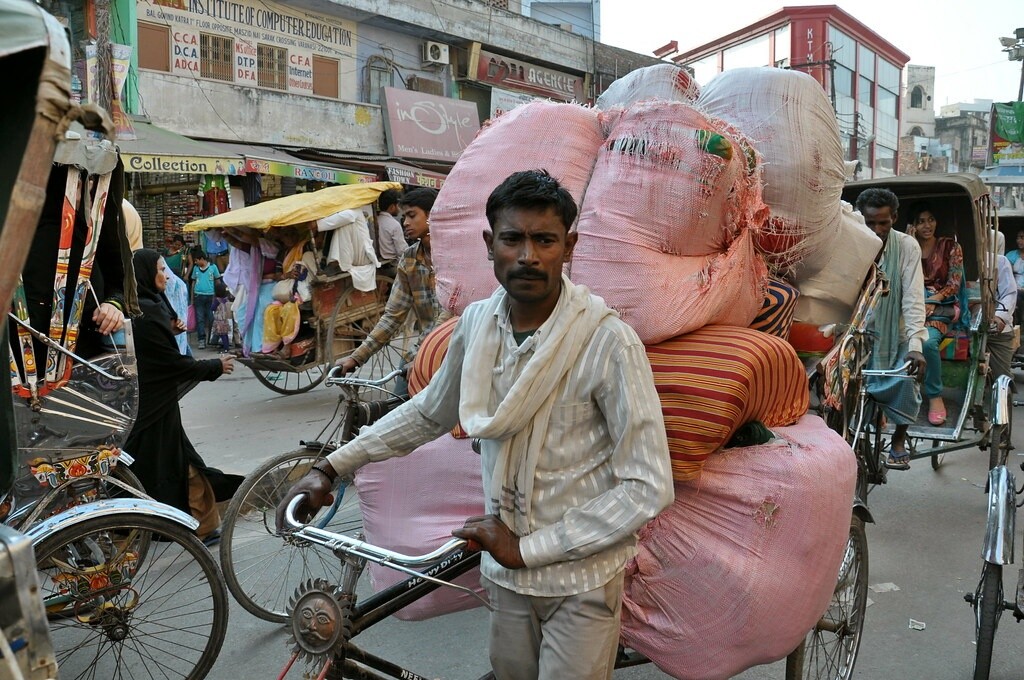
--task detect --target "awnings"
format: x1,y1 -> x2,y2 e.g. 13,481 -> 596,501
109,120 -> 376,184
310,154 -> 447,188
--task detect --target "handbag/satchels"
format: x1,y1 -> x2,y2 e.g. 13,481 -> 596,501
187,304 -> 197,334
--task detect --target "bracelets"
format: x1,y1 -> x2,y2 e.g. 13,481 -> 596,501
312,465 -> 333,484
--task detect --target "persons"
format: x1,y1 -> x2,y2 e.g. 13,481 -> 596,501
275,171 -> 672,680
377,189 -> 409,259
334,189 -> 439,397
216,208 -> 375,360
120,248 -> 249,548
190,247 -> 221,350
851,188 -> 1024,467
10,169 -> 144,398
161,234 -> 193,285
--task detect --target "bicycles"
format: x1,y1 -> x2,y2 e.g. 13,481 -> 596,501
220,357 -> 410,625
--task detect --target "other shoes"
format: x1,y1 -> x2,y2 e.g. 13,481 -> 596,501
202,529 -> 221,546
199,339 -> 206,349
927,408 -> 946,425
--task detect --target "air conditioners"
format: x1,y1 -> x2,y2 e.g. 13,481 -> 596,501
423,39 -> 451,67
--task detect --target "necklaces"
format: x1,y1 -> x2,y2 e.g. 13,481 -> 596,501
424,247 -> 432,261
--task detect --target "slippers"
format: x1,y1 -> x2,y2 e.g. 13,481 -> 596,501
886,448 -> 910,468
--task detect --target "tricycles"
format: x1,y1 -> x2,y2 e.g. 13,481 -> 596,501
832,173 -> 1024,592
180,180 -> 406,397
964,463 -> 1024,680
275,260 -> 889,680
1,0 -> 230,679
